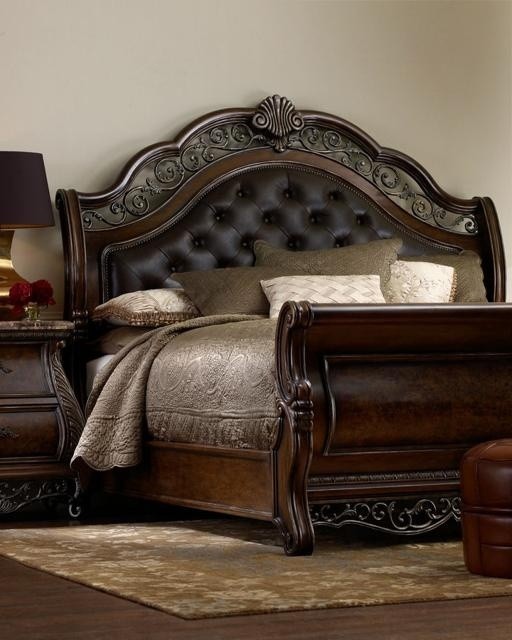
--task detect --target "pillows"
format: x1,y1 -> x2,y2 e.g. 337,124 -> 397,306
91,287 -> 202,328
399,250 -> 488,303
101,326 -> 156,353
388,260 -> 458,303
259,274 -> 387,320
254,237 -> 404,302
169,267 -> 276,316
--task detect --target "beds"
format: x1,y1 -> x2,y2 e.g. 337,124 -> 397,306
54,93 -> 511,556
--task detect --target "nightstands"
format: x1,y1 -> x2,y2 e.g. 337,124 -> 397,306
0,319 -> 87,520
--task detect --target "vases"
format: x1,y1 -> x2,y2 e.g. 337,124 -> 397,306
27,302 -> 41,319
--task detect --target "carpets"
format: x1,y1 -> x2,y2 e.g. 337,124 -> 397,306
0,514 -> 511,620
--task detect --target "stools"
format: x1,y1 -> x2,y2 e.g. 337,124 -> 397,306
460,438 -> 512,579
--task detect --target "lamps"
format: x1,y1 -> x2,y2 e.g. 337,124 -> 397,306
0,151 -> 56,319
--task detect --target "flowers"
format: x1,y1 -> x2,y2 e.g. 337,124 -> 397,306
9,279 -> 56,319
